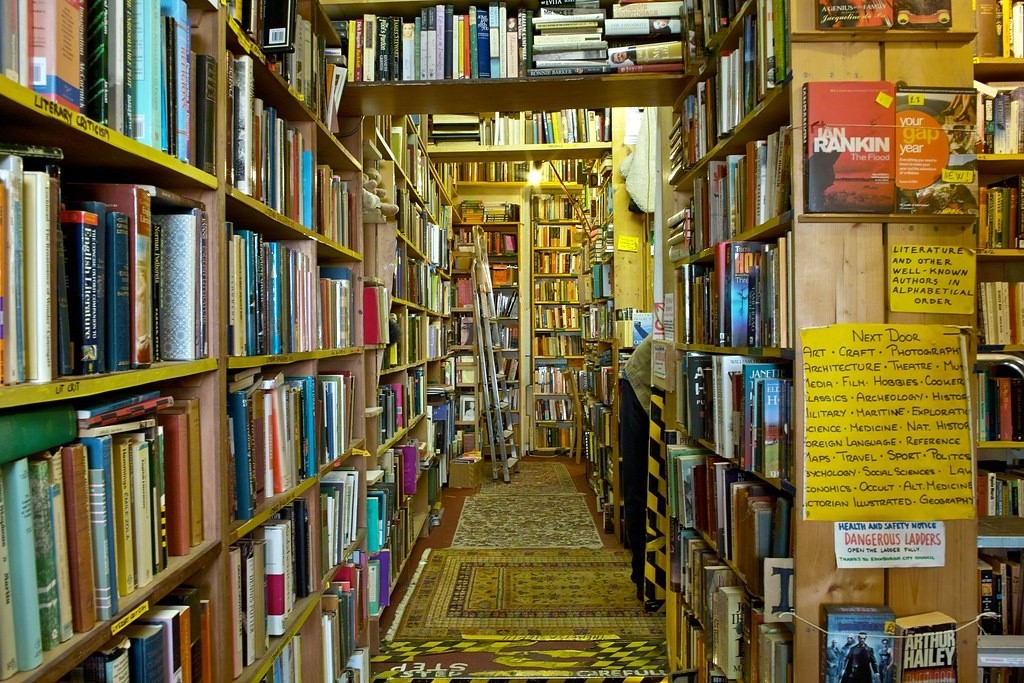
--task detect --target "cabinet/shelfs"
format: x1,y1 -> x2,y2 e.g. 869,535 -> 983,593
0,0 -> 1024,683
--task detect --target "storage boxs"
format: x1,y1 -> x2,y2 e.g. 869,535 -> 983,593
448,455 -> 485,489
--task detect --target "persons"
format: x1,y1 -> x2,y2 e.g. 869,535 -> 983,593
618,333 -> 651,602
827,632 -> 894,683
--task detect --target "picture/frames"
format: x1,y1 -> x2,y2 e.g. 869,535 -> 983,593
459,394 -> 475,422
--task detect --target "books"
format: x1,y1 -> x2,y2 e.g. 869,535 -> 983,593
976,0 -> 1024,683
897,0 -> 954,30
802,81 -> 896,214
1,1 -> 794,683
894,611 -> 961,683
818,0 -> 896,32
895,87 -> 980,217
820,604 -> 896,683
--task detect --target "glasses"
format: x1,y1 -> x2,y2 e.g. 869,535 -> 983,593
883,642 -> 888,645
860,638 -> 866,640
847,637 -> 853,639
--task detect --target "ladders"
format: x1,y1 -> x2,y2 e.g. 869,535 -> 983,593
469,223 -> 521,483
